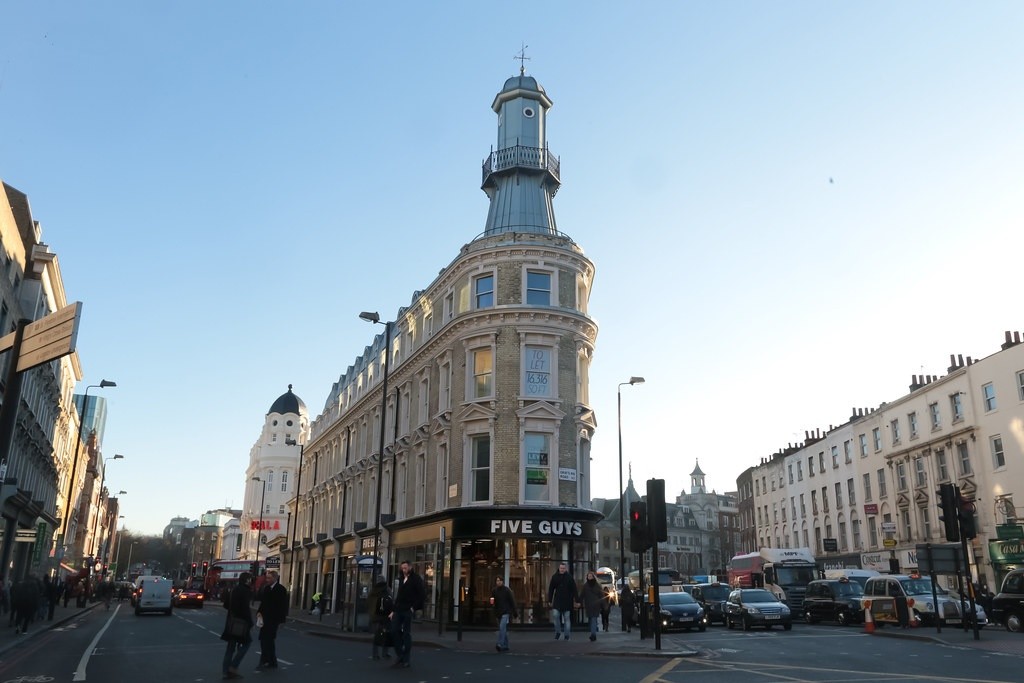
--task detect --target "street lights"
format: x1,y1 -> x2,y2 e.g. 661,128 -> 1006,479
49,380 -> 117,621
91,453 -> 127,558
125,541 -> 139,581
618,376 -> 646,589
116,529 -> 129,562
286,438 -> 305,582
252,475 -> 266,562
360,311 -> 390,633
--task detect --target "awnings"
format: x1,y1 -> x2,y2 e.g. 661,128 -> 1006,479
60,563 -> 77,574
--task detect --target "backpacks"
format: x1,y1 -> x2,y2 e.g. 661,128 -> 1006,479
376,595 -> 393,617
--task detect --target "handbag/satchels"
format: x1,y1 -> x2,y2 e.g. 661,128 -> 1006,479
228,618 -> 248,638
312,607 -> 320,616
375,618 -> 395,647
256,614 -> 263,631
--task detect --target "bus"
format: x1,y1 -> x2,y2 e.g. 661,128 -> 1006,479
597,567 -> 617,604
204,559 -> 279,601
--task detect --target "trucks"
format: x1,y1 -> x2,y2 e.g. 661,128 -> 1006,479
729,551 -> 826,621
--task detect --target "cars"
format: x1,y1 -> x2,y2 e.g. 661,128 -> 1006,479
172,588 -> 204,608
633,568 -> 1023,634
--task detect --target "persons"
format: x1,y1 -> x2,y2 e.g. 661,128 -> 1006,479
117,588 -> 124,601
599,587 -> 615,633
574,571 -> 607,642
389,560 -> 424,669
548,563 -> 580,642
906,584 -> 915,595
105,582 -> 116,609
220,572 -> 256,679
618,585 -> 635,633
8,574 -> 72,635
256,571 -> 288,669
489,575 -> 518,651
369,575 -> 393,660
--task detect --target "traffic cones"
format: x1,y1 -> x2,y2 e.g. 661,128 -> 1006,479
908,600 -> 920,628
861,607 -> 876,634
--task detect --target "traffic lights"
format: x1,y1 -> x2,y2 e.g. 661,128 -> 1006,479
631,501 -> 645,551
935,483 -> 958,540
203,562 -> 207,577
192,562 -> 197,577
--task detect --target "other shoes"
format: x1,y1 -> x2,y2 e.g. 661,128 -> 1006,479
257,664 -> 277,672
589,636 -> 595,641
390,661 -> 410,669
224,665 -> 244,681
496,644 -> 509,653
16,625 -> 19,634
554,632 -> 569,640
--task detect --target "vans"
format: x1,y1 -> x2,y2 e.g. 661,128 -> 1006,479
115,576 -> 173,616
